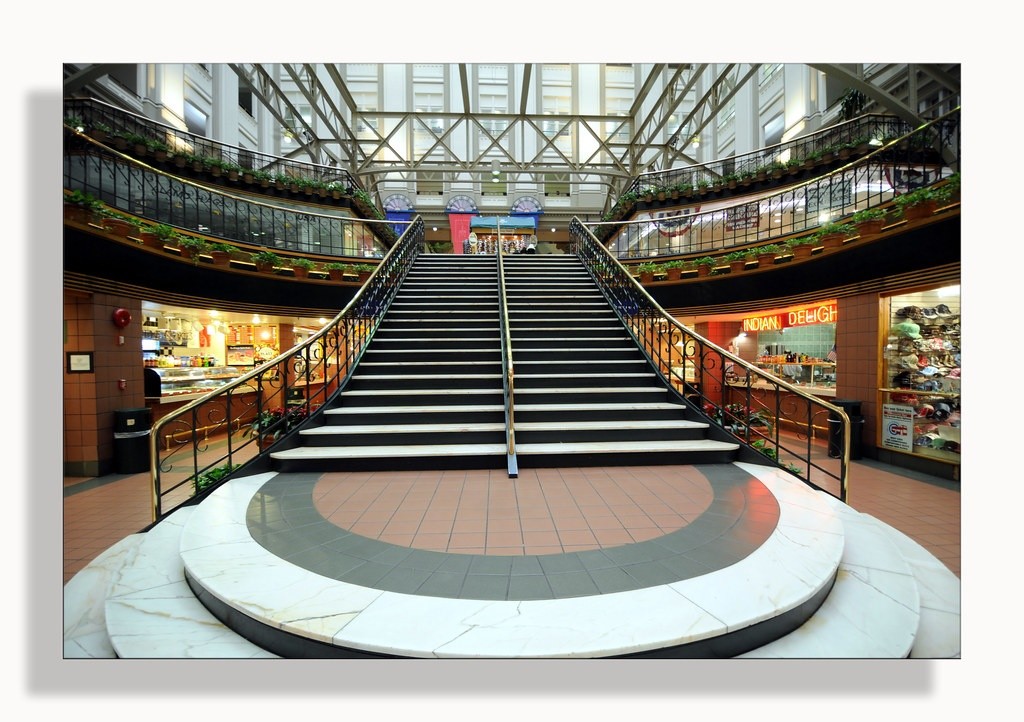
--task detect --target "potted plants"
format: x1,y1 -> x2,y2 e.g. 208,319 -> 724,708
582,132 -> 961,283
63,116 -> 398,284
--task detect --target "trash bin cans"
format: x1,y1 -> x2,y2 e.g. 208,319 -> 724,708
826,400 -> 865,461
683,382 -> 700,410
286,386 -> 305,408
110,408 -> 154,474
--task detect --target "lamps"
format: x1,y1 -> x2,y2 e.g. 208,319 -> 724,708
144,317 -> 231,335
692,135 -> 700,148
283,130 -> 293,143
491,159 -> 500,184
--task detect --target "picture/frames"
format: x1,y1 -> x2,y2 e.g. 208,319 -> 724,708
66,351 -> 94,374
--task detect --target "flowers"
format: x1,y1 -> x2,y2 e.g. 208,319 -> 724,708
235,404 -> 319,446
701,402 -> 774,442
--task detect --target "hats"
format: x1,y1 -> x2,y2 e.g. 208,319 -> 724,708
917,398 -> 961,420
893,304 -> 961,390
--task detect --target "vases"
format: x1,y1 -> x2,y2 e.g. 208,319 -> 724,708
725,426 -> 776,444
252,434 -> 283,453
